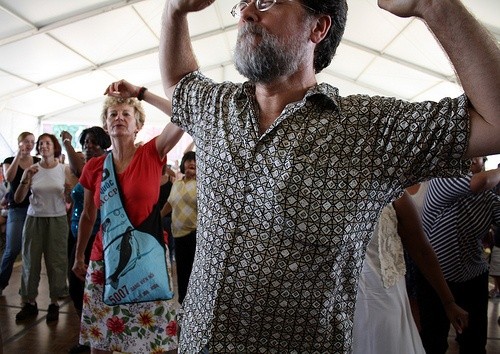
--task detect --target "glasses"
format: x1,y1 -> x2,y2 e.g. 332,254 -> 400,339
231,0 -> 278,12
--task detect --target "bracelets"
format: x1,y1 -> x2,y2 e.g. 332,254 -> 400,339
443,300 -> 455,306
62,138 -> 70,145
138,86 -> 148,101
20,180 -> 28,184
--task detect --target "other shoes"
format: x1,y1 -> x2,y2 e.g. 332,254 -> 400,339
47,303 -> 58,323
17,302 -> 37,321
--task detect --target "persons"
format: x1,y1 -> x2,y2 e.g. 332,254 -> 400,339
0,126 -> 500,354
71,80 -> 185,354
157,0 -> 500,354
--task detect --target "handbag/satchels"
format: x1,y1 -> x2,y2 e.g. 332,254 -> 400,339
101,152 -> 173,304
